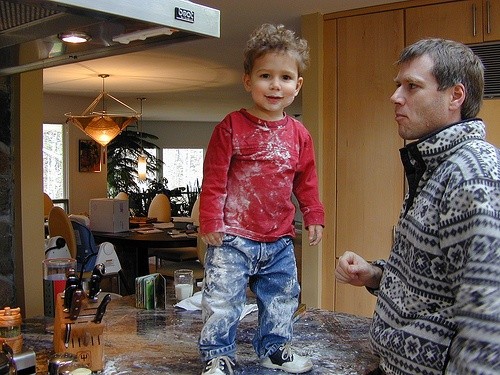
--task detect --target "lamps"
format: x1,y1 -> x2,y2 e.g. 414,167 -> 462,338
57,29 -> 93,43
137,97 -> 147,180
64,74 -> 148,165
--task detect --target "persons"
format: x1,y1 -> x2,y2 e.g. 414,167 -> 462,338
199,24 -> 324,375
333,37 -> 500,375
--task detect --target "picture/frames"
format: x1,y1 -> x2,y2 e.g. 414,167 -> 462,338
78,139 -> 102,173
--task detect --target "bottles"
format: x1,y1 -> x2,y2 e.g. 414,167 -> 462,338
41,257 -> 78,334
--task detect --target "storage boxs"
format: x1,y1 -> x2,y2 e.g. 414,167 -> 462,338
90,198 -> 130,234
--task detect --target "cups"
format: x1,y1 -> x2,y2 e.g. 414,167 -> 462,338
174,268 -> 194,302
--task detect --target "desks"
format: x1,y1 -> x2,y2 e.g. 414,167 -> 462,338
44,217 -> 200,297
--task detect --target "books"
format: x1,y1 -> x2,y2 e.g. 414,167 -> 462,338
169,232 -> 198,238
132,226 -> 164,234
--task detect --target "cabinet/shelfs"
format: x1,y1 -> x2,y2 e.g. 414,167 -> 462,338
323,0 -> 500,319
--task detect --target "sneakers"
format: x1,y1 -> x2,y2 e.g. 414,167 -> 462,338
260,344 -> 312,373
201,356 -> 236,375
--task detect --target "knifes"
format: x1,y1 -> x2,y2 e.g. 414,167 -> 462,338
63,261 -> 112,345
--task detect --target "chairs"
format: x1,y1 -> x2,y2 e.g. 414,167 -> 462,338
43,192 -> 209,284
